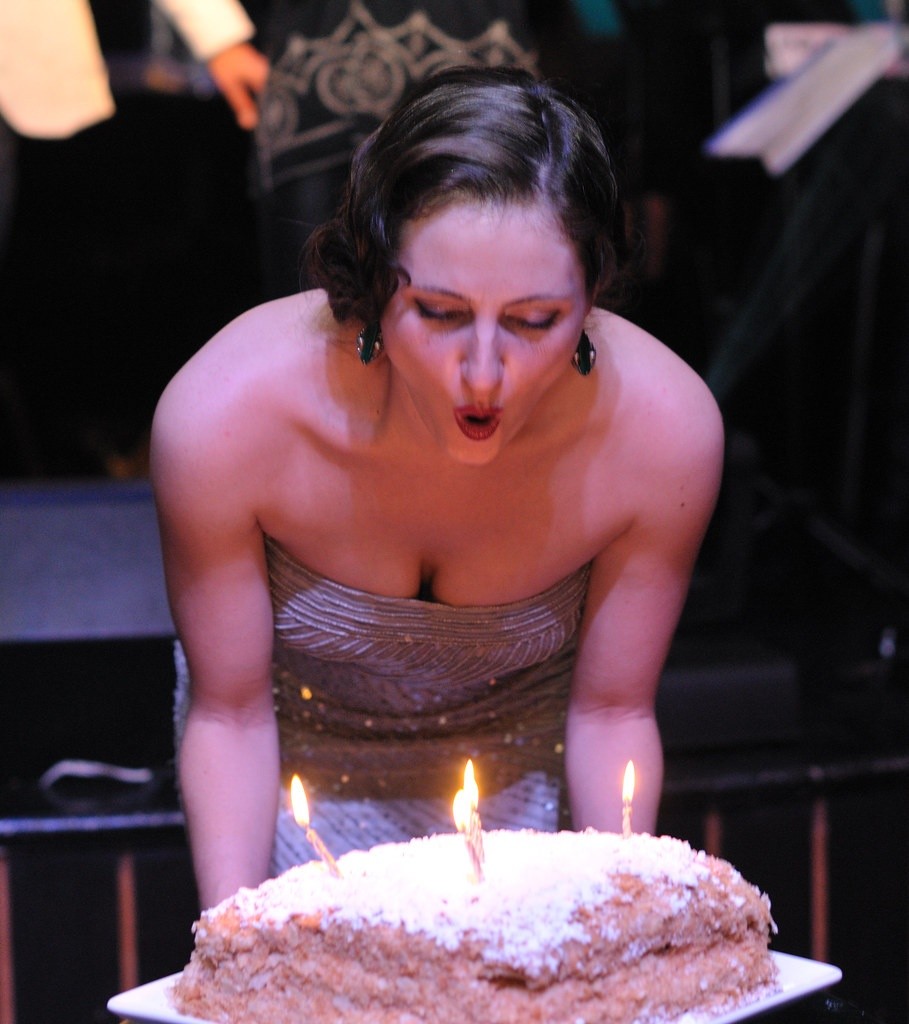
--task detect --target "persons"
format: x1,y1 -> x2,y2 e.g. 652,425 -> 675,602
149,64 -> 727,915
0,0 -> 269,482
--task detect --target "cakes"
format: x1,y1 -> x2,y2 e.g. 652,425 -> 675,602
167,828 -> 780,1024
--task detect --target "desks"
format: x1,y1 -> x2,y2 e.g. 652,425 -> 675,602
108,948 -> 843,1024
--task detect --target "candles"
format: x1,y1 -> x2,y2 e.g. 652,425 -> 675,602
452,788 -> 486,882
290,774 -> 345,880
623,757 -> 636,838
463,758 -> 486,864
